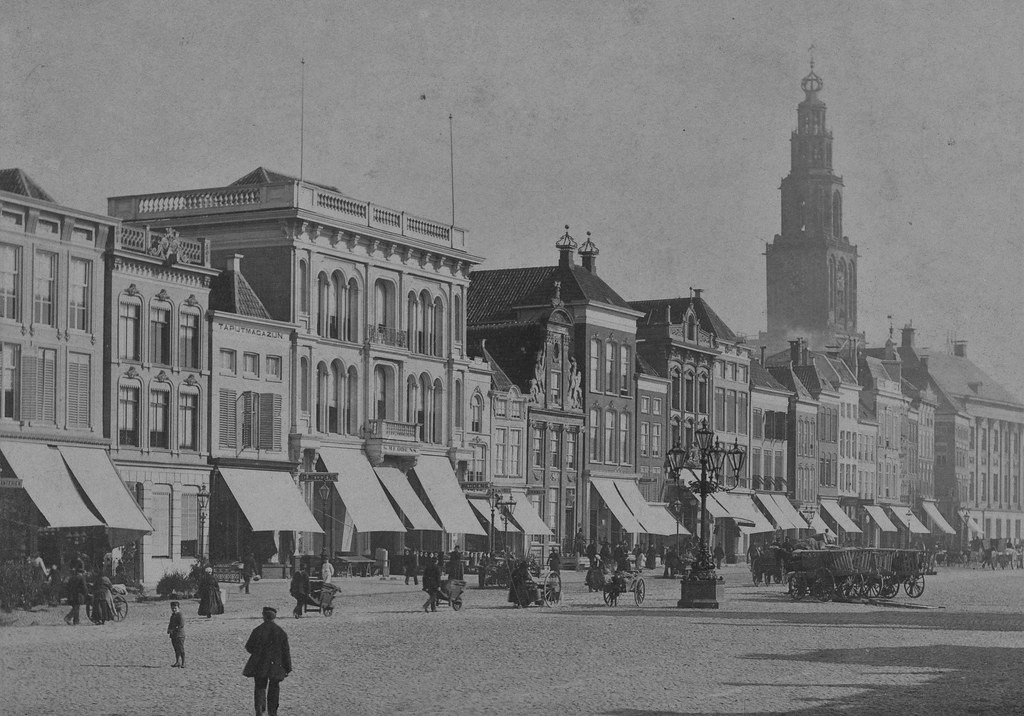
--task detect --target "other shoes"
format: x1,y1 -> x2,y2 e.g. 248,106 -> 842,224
423,605 -> 428,612
432,609 -> 437,612
64,617 -> 72,625
171,663 -> 180,667
181,664 -> 184,668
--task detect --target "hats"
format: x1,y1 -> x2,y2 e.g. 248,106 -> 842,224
261,607 -> 277,614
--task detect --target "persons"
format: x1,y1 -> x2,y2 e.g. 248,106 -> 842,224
243,607 -> 293,716
319,546 -> 339,577
167,601 -> 186,669
576,527 -> 725,607
193,566 -> 225,617
291,556 -> 337,619
239,552 -> 258,594
0,540 -> 138,625
747,528 -> 1014,587
403,545 -> 562,613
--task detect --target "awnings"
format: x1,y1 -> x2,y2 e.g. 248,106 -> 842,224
959,509 -> 983,534
862,504 -> 897,532
890,505 -> 931,534
694,491 -> 775,535
222,465 -> 325,534
372,455 -> 487,536
468,491 -> 554,535
819,499 -> 863,533
592,477 -> 691,536
755,492 -> 839,540
922,501 -> 956,535
0,441 -> 157,532
319,446 -> 408,533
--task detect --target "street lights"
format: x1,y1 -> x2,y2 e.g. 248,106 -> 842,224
494,488 -> 518,555
961,511 -> 970,562
673,496 -> 683,558
318,481 -> 331,580
905,509 -> 914,548
197,484 -> 212,572
663,416 -> 749,610
484,487 -> 503,555
801,504 -> 816,537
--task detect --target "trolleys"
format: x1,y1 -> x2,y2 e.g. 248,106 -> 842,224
422,579 -> 467,611
601,569 -> 646,607
84,588 -> 130,625
509,571 -> 563,608
301,577 -> 343,617
746,546 -> 935,603
